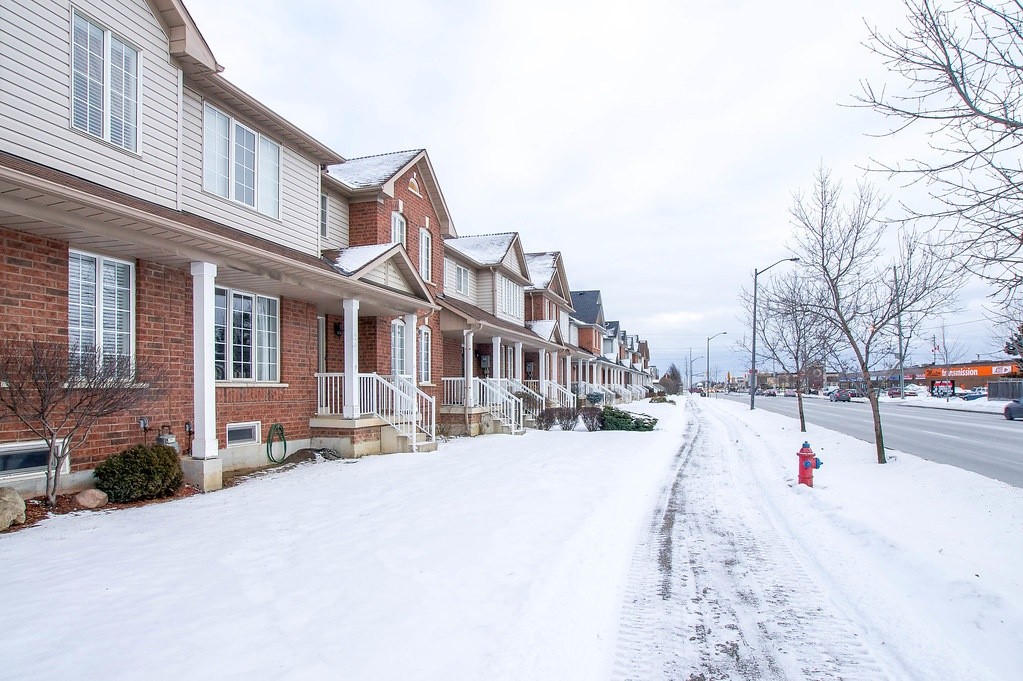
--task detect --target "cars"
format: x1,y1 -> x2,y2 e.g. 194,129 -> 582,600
763,389 -> 776,397
784,389 -> 796,397
829,388 -> 852,402
689,380 -> 819,397
822,385 -> 887,398
932,386 -> 988,401
1004,395 -> 1023,420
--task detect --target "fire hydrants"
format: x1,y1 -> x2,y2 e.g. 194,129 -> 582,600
797,440 -> 823,488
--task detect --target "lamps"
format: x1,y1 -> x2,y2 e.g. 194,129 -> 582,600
475,349 -> 480,359
334,321 -> 343,338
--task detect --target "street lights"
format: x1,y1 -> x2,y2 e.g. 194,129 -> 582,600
750,258 -> 801,411
689,347 -> 703,395
705,332 -> 727,397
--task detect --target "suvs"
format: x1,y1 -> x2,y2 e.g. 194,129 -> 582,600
888,387 -> 918,398
